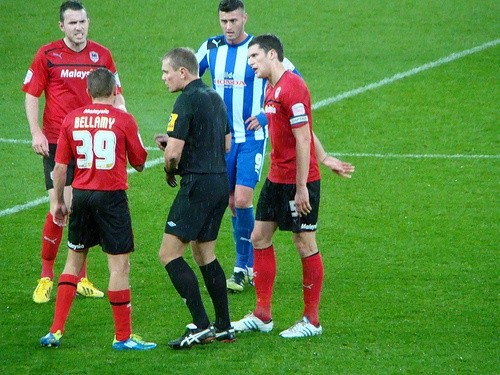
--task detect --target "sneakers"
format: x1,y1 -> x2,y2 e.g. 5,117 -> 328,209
225,271 -> 254,293
279,315 -> 323,338
33,277 -> 53,304
168,323 -> 215,349
112,334 -> 157,350
212,324 -> 235,343
76,273 -> 104,297
40,329 -> 64,348
230,312 -> 274,333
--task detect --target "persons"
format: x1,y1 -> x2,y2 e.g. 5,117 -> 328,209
40,67 -> 157,349
193,0 -> 302,292
229,34 -> 322,338
153,47 -> 237,348
21,1 -> 127,304
